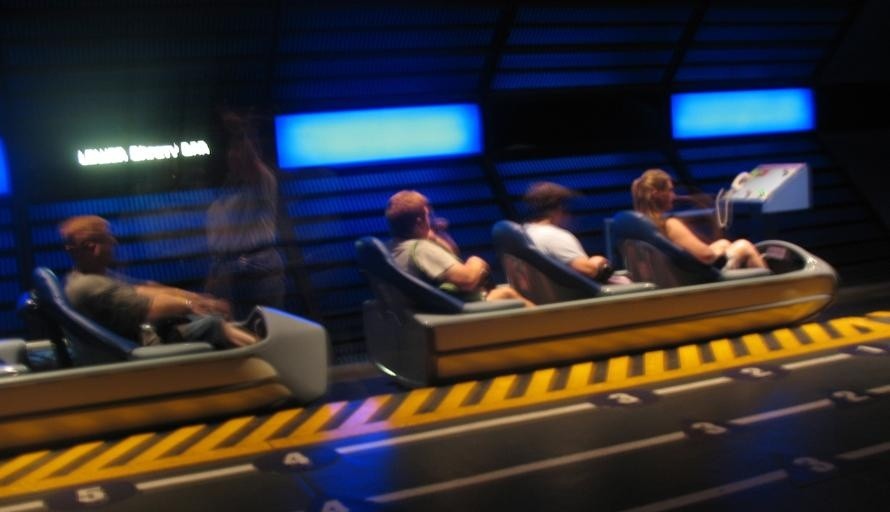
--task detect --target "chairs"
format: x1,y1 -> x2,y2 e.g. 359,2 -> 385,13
494,220 -> 656,305
354,238 -> 526,318
34,269 -> 136,366
609,211 -> 769,289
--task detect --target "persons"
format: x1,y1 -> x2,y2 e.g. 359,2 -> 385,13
386,183 -> 540,311
202,104 -> 289,322
629,163 -> 769,277
52,213 -> 261,367
522,176 -> 632,289
